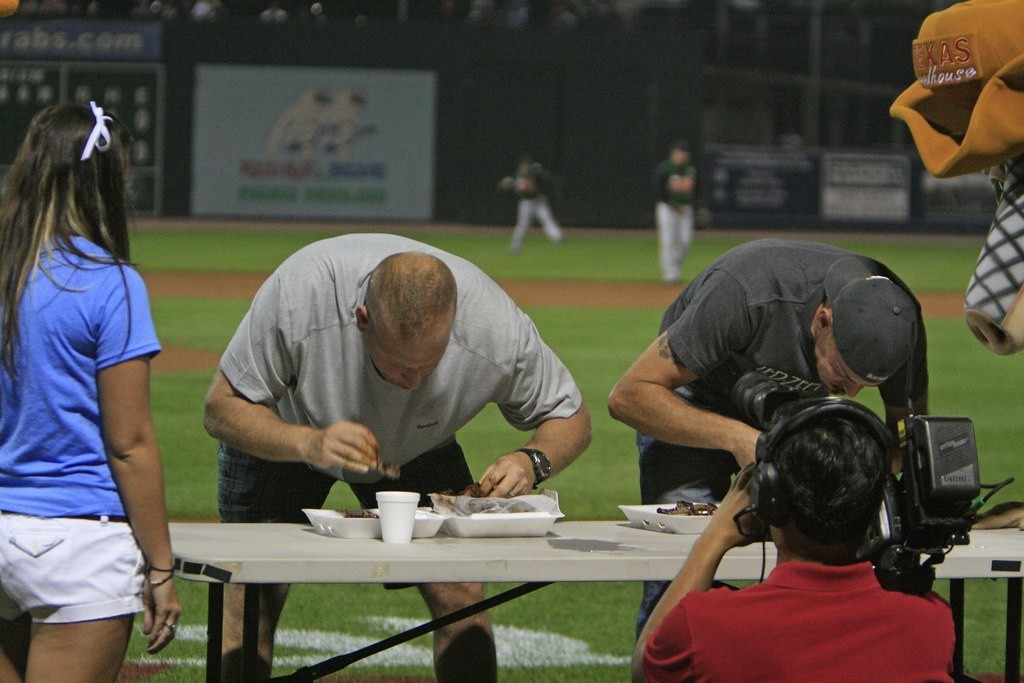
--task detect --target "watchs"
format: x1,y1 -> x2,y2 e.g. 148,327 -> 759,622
514,448 -> 551,490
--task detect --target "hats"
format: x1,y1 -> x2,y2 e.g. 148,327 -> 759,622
831,275 -> 921,384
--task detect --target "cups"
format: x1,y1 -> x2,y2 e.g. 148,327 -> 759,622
376,491 -> 420,544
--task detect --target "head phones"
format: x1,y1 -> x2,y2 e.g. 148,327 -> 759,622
748,396 -> 893,528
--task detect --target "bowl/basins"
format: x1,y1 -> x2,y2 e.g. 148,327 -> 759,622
301,507 -> 565,539
617,501 -> 720,535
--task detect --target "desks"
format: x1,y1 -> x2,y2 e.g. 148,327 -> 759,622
166,513 -> 1024,683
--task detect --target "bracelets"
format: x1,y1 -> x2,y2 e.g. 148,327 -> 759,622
147,563 -> 173,587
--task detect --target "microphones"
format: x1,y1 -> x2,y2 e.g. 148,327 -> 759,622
734,503 -> 766,542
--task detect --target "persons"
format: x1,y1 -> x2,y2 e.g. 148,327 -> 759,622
631,396 -> 956,683
501,152 -> 564,256
654,138 -> 698,284
203,234 -> 594,683
607,236 -> 929,645
0,102 -> 183,683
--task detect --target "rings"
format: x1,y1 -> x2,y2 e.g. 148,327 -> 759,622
164,623 -> 177,633
